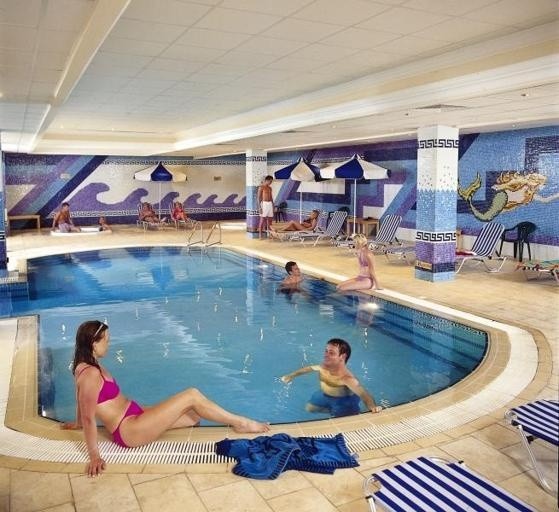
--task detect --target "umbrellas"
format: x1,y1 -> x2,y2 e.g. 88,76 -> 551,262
320,153 -> 391,235
275,157 -> 331,225
134,162 -> 188,220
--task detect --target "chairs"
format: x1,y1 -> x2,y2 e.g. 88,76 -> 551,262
346,215 -> 404,262
500,222 -> 536,262
364,455 -> 540,512
453,223 -> 507,273
265,207 -> 348,247
136,205 -> 166,233
169,207 -> 197,230
517,259 -> 559,283
504,399 -> 559,499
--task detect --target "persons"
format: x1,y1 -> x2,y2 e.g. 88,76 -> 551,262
282,339 -> 383,416
278,210 -> 319,230
258,176 -> 274,238
139,203 -> 166,223
51,203 -> 81,233
352,292 -> 375,328
173,202 -> 187,221
336,234 -> 384,291
278,261 -> 318,286
458,172 -> 559,222
99,216 -> 111,231
60,321 -> 272,478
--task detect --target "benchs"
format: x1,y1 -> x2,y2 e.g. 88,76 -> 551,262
7,215 -> 40,229
